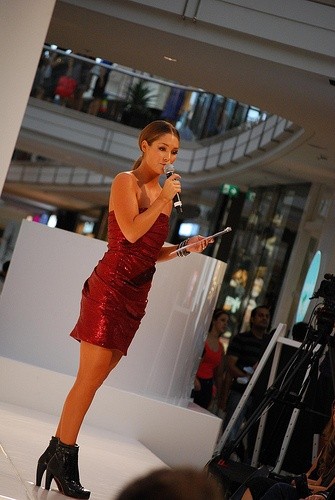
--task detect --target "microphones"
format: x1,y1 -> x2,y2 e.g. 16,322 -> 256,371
163,164 -> 183,214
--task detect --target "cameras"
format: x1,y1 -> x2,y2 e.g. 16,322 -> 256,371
295,473 -> 308,493
314,273 -> 335,310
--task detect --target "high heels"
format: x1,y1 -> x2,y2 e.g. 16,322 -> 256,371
36,436 -> 65,494
45,438 -> 91,500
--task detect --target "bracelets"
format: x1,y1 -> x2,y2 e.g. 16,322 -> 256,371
176,238 -> 191,257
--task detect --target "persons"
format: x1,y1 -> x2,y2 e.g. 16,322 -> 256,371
191,308 -> 230,410
30,42 -> 96,112
116,402 -> 335,500
86,60 -> 114,116
222,305 -> 271,461
36,120 -> 214,500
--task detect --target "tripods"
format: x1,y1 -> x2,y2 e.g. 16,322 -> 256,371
222,329 -> 335,464
